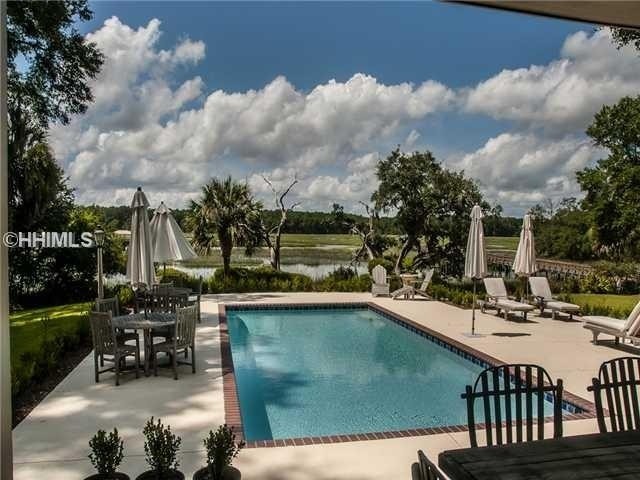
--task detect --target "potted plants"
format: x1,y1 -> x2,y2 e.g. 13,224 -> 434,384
84,415 -> 246,480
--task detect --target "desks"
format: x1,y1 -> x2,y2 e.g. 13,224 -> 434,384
437,428 -> 639,480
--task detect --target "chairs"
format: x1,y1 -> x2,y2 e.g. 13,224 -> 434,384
89,275 -> 204,387
582,302 -> 639,346
477,276 -> 581,323
460,357 -> 639,449
370,264 -> 435,300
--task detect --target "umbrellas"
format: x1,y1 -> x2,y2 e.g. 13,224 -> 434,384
512,216 -> 538,275
464,202 -> 489,278
128,187 -> 156,293
151,201 -> 199,287
